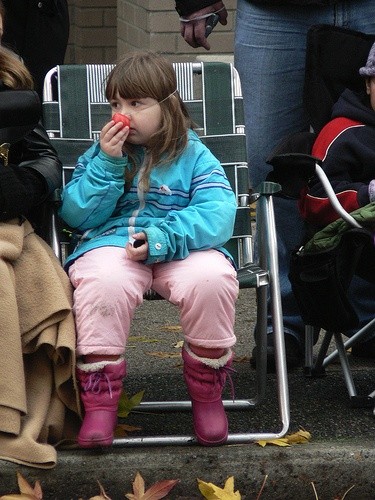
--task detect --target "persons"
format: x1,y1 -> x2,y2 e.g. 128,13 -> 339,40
56,50 -> 238,449
176,0 -> 375,369
292,35 -> 375,370
0,46 -> 84,445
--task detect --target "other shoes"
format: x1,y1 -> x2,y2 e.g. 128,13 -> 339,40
250,328 -> 301,371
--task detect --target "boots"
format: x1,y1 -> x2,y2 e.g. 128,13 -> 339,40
182,339 -> 237,446
76,358 -> 127,449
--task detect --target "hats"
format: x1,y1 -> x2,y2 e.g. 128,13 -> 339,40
359,42 -> 375,78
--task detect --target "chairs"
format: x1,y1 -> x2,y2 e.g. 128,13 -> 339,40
264,21 -> 375,410
42,61 -> 289,447
